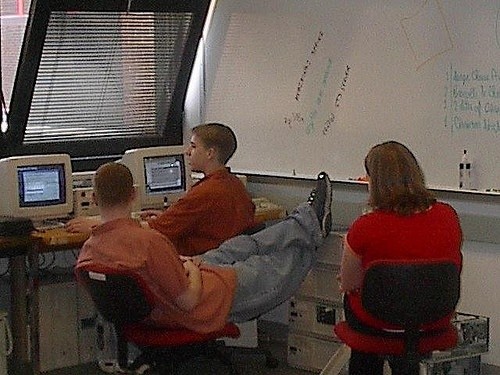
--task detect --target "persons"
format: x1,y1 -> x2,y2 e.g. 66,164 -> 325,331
73,162 -> 333,350
64,123 -> 256,374
340,140 -> 465,375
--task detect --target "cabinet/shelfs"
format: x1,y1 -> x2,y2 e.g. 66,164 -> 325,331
32,270 -> 113,373
286,232 -> 391,374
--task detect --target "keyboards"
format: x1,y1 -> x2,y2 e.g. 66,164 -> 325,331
128,210 -> 166,231
0,224 -> 90,254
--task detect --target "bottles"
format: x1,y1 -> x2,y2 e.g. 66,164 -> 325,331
458,149 -> 471,190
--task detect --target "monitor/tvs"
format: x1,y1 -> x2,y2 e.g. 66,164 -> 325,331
0,154 -> 74,230
113,145 -> 191,211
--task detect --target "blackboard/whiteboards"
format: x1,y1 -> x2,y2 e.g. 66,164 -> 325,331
200,0 -> 500,195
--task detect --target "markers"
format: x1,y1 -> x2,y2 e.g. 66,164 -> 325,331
466,163 -> 472,191
458,163 -> 465,190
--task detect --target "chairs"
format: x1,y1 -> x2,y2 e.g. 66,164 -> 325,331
76,262 -> 280,375
333,261 -> 461,375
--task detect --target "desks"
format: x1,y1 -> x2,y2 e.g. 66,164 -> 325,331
0,202 -> 285,375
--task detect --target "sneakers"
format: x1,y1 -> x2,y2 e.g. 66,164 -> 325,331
308,171 -> 332,238
